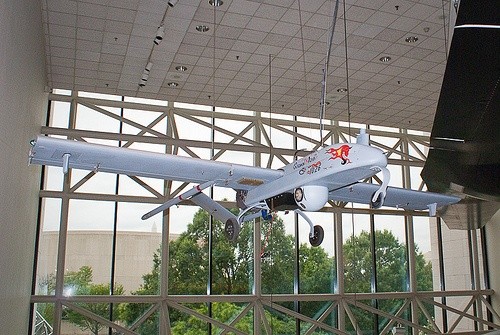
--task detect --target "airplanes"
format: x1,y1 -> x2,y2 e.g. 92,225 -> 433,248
24,127 -> 462,247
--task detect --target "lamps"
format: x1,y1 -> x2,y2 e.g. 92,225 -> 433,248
167,0 -> 179,8
138,80 -> 146,86
153,26 -> 165,45
144,62 -> 154,74
141,74 -> 148,81
